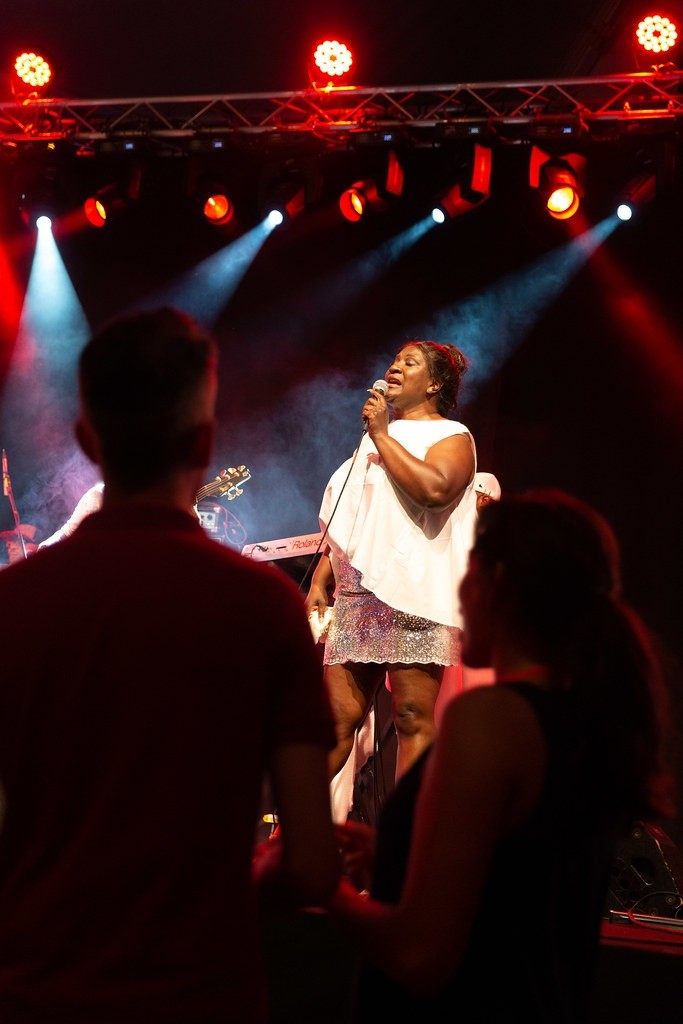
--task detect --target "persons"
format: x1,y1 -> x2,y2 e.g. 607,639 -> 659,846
0,301 -> 346,1024
338,488 -> 670,1023
303,341 -> 478,785
476,472 -> 501,511
0,479 -> 106,564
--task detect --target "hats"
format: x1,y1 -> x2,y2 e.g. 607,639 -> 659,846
0,524 -> 44,545
475,472 -> 501,502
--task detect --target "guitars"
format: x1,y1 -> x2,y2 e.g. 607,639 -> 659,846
192,463 -> 253,508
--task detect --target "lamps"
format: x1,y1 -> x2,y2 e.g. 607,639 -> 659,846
608,168 -> 658,225
534,157 -> 582,221
13,142 -> 490,235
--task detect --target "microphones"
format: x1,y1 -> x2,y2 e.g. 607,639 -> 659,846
3,448 -> 10,495
361,380 -> 389,433
